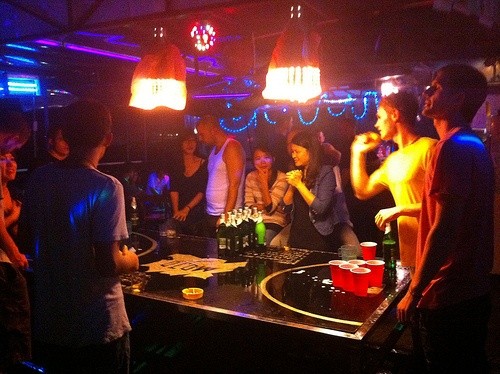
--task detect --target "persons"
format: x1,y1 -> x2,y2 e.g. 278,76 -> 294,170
197,113 -> 246,238
280,132 -> 337,251
246,144 -> 291,246
267,64 -> 495,374
24,125 -> 69,170
0,97 -> 139,374
158,133 -> 207,236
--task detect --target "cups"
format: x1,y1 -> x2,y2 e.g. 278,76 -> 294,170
165,220 -> 176,238
348,259 -> 366,267
329,260 -> 347,287
350,267 -> 371,297
126,221 -> 132,235
360,242 -> 377,260
159,223 -> 166,236
339,263 -> 359,292
366,260 -> 385,288
338,244 -> 357,260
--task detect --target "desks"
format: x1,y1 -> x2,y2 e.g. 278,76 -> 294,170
120,228 -> 411,374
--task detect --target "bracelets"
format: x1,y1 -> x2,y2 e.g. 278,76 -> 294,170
185,206 -> 192,212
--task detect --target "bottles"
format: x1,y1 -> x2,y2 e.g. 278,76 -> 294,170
217,205 -> 266,257
382,221 -> 395,268
130,197 -> 139,225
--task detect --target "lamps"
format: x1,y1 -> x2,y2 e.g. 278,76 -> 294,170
262,21 -> 324,102
129,42 -> 188,111
